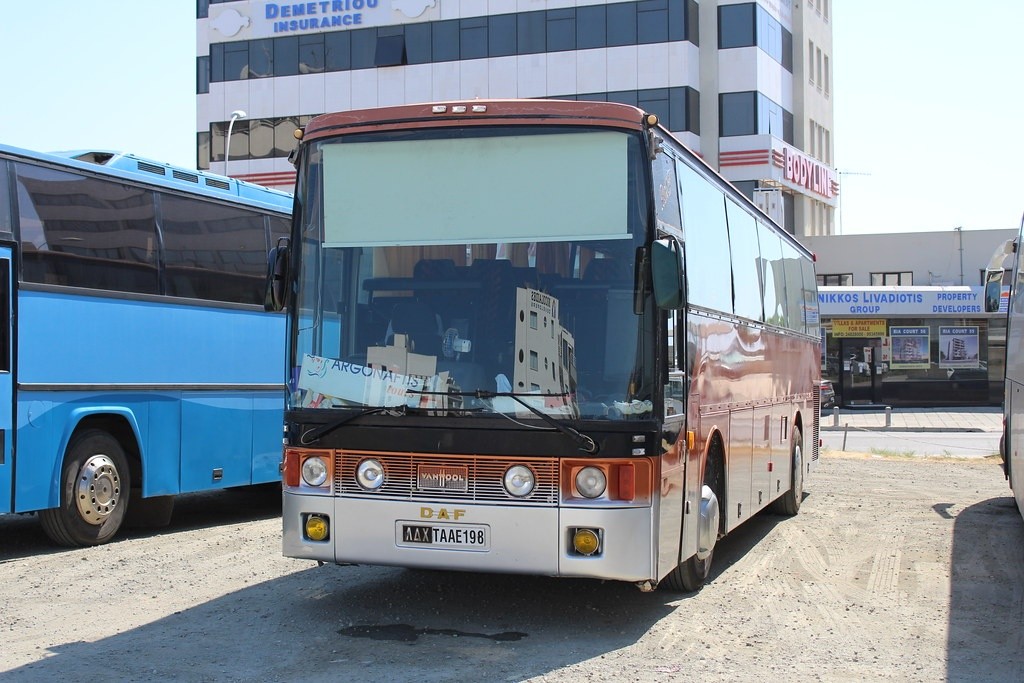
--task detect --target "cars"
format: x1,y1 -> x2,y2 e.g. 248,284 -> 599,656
820,375 -> 834,410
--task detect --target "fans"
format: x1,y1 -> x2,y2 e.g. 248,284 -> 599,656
442,328 -> 472,361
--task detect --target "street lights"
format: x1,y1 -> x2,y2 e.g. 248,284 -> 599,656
224,110 -> 248,173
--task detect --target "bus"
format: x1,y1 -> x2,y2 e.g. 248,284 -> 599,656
984,210 -> 1024,521
0,143 -> 390,553
264,97 -> 827,605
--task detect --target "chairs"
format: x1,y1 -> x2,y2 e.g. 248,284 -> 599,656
367,258 -> 628,383
